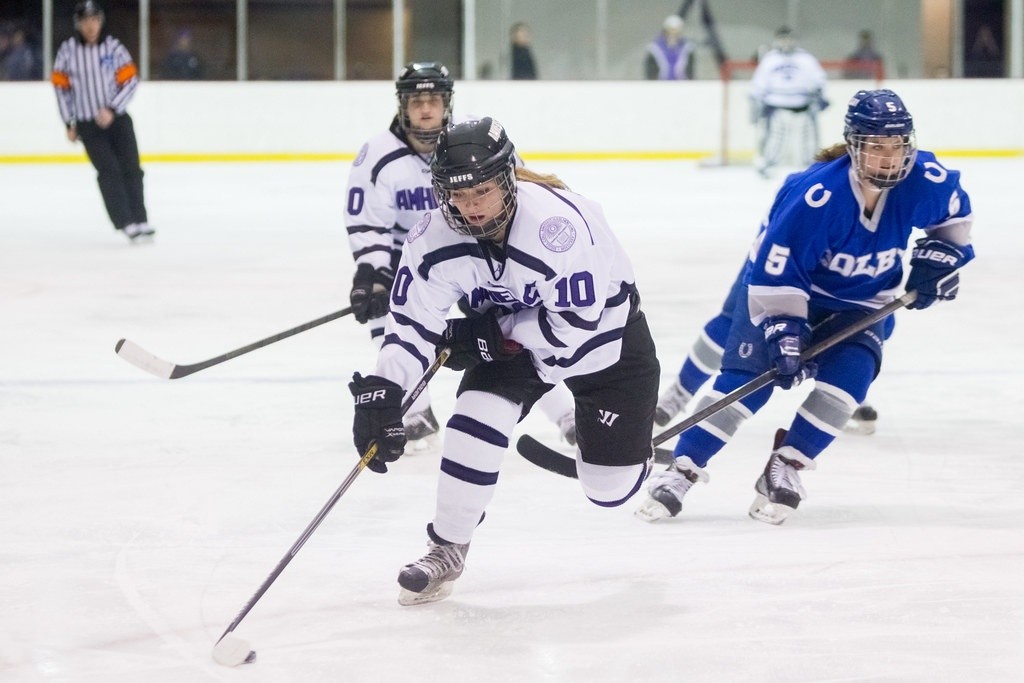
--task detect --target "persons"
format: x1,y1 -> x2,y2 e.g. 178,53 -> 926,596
745,27 -> 835,181
162,30 -> 206,81
654,172 -> 895,435
643,14 -> 695,80
0,28 -> 55,81
348,118 -> 660,607
634,89 -> 972,525
52,0 -> 155,241
841,29 -> 884,81
972,25 -> 1003,79
511,22 -> 540,81
344,62 -> 576,457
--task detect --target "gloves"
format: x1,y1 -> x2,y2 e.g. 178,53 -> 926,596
434,318 -> 523,371
762,315 -> 818,389
350,372 -> 407,474
350,264 -> 396,324
904,237 -> 974,310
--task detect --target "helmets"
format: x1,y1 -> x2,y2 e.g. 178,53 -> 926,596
395,61 -> 453,143
844,90 -> 917,189
74,1 -> 108,45
431,117 -> 518,238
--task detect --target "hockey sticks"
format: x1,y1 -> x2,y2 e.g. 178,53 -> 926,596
114,300 -> 360,381
211,343 -> 452,668
516,288 -> 919,480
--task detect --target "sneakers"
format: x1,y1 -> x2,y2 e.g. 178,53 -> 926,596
846,408 -> 879,430
397,511 -> 486,605
401,407 -> 444,455
551,403 -> 577,446
124,224 -> 142,244
749,428 -> 816,525
142,222 -> 155,238
654,378 -> 695,428
632,455 -> 708,522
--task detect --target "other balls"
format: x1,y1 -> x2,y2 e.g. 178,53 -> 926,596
244,650 -> 257,664
